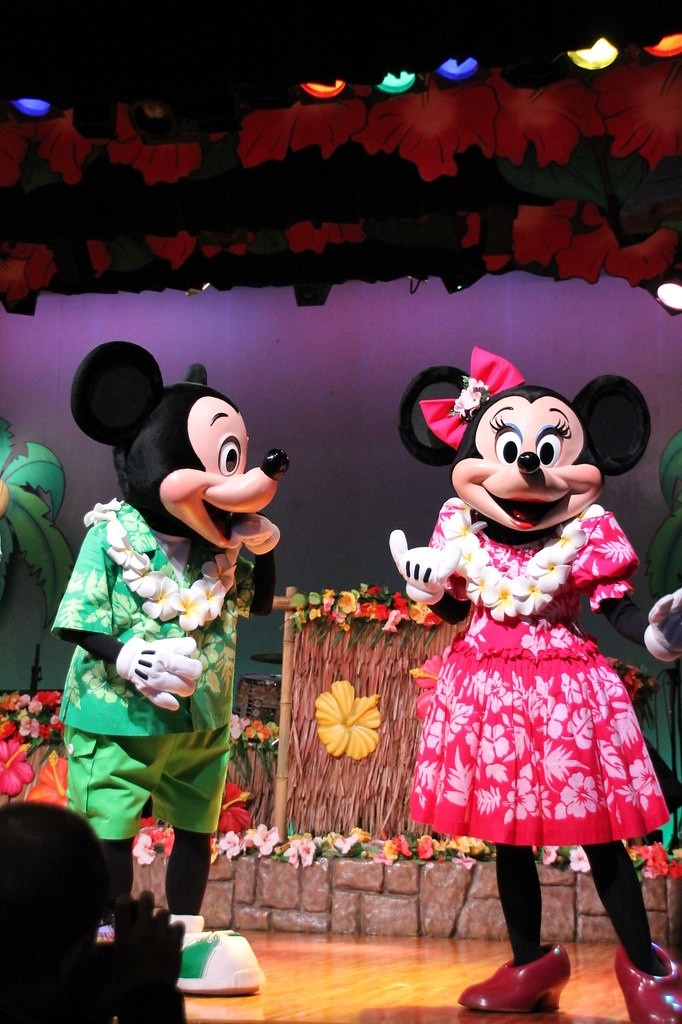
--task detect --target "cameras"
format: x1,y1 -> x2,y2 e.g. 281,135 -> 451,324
94,914 -> 115,945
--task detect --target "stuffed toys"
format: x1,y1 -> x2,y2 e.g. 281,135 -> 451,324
47,340 -> 288,995
386,344 -> 681,1024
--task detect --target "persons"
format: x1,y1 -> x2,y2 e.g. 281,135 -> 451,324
0,802 -> 189,1024
